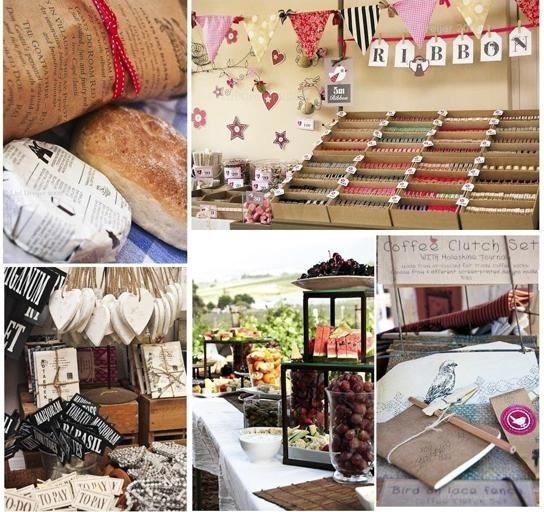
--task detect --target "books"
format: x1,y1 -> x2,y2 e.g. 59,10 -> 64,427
378,403 -> 501,490
78,346 -> 121,389
24,335 -> 79,411
376,478 -> 536,507
128,341 -> 187,400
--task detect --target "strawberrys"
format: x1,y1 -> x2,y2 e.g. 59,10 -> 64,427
304,417 -> 312,424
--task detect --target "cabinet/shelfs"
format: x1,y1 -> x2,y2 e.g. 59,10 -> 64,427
129,381 -> 186,446
18,383 -> 139,455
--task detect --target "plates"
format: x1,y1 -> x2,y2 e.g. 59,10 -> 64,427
238,386 -> 282,398
287,446 -> 335,464
239,425 -> 282,434
291,274 -> 374,288
192,391 -> 237,398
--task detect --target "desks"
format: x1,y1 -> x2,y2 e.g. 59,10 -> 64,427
203,338 -> 273,387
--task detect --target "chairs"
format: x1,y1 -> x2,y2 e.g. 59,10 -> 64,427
191,409 -> 258,511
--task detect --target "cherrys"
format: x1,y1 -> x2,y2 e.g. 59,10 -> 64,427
300,253 -> 374,278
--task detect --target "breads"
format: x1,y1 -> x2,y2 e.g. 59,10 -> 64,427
68,103 -> 188,254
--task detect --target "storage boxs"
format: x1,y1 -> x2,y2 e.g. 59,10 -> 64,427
366,144 -> 423,157
477,154 -> 538,173
404,171 -> 472,186
346,170 -> 409,183
356,155 -> 415,173
496,110 -> 537,121
440,112 -> 495,121
473,170 -> 539,187
489,132 -> 538,144
271,195 -> 331,223
427,132 -> 484,144
421,145 -> 483,155
386,111 -> 438,124
339,112 -> 386,123
463,185 -> 538,202
493,121 -> 539,133
379,123 -> 436,134
373,134 -> 430,145
398,183 -> 466,208
459,199 -> 538,230
301,156 -> 356,171
288,171 -> 346,182
390,198 -> 461,230
328,195 -> 392,227
324,133 -> 374,142
312,142 -> 366,153
284,182 -> 335,195
333,123 -> 380,131
482,144 -> 539,156
337,182 -> 400,197
413,157 -> 478,172
435,121 -> 490,134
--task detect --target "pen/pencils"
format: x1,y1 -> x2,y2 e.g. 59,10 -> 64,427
407,396 -> 517,455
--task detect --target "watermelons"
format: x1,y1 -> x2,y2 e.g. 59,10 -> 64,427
307,325 -> 374,363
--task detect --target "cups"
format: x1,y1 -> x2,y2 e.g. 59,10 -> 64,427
323,387 -> 378,483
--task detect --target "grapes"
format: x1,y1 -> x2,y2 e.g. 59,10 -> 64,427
328,372 -> 374,478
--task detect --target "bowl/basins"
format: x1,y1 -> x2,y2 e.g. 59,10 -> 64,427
238,434 -> 283,464
